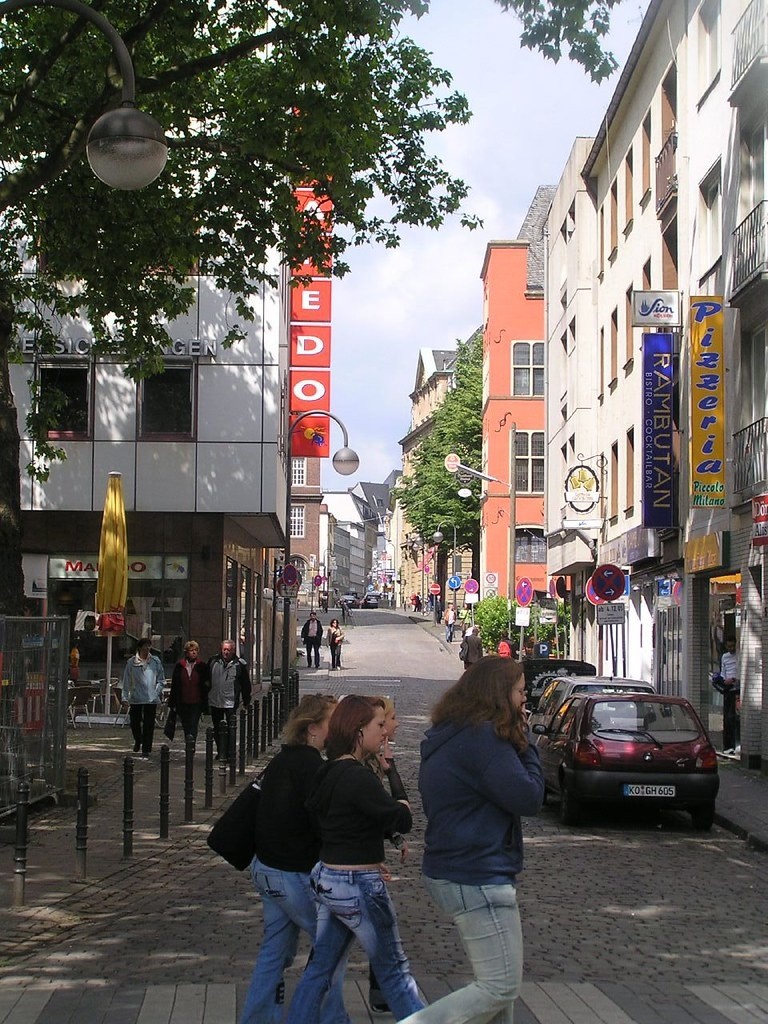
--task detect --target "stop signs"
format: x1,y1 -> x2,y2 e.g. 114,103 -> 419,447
430,584 -> 440,595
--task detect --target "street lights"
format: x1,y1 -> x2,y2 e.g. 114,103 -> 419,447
433,520 -> 456,606
280,409 -> 360,728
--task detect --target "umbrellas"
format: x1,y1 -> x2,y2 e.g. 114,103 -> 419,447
47,610 -> 101,631
94,469 -> 127,715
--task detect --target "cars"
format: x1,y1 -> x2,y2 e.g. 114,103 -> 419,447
530,693 -> 721,829
524,674 -> 663,748
334,591 -> 360,609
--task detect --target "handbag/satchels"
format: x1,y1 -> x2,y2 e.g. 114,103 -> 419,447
459,636 -> 469,661
207,766 -> 268,872
163,708 -> 177,742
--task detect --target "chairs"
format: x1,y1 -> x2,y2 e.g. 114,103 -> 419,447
47,675 -> 174,728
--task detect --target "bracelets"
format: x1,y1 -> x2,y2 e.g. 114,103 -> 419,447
732,677 -> 735,682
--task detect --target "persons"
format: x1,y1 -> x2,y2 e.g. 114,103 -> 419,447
411,591 -> 433,616
719,636 -> 736,754
436,595 -> 444,624
460,629 -> 483,670
285,696 -> 425,1024
121,638 -> 165,756
459,603 -> 472,637
305,695 -> 408,1014
464,624 -> 481,638
167,641 -> 212,737
444,602 -> 457,642
495,631 -> 534,658
301,612 -> 323,668
394,656 -> 545,1023
326,619 -> 344,670
207,640 -> 252,759
239,693 -> 391,1024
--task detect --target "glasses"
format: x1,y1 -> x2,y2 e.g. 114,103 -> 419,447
512,687 -> 528,700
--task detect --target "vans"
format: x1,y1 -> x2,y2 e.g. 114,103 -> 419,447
516,659 -> 597,715
361,594 -> 381,609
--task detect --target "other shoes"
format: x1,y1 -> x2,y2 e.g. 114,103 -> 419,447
307,665 -> 311,668
332,668 -> 336,670
142,753 -> 150,756
315,666 -> 318,668
371,1003 -> 391,1013
723,748 -> 735,754
336,666 -> 340,671
133,742 -> 141,752
